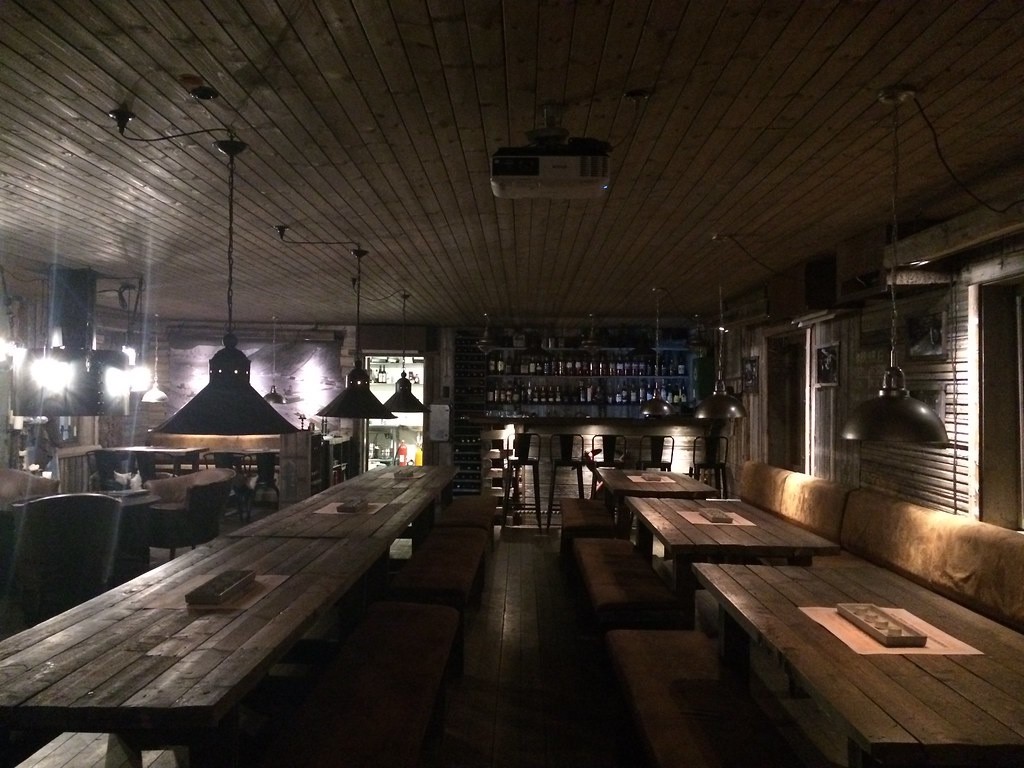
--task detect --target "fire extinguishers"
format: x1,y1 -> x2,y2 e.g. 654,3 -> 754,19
397,440 -> 407,467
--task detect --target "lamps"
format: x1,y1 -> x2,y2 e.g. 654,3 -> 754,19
473,290 -> 761,421
832,87 -> 955,445
1,98 -> 429,436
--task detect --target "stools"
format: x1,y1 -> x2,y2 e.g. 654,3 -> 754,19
504,432 -> 729,534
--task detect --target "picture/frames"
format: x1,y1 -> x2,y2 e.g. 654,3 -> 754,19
738,356 -> 759,392
816,342 -> 841,390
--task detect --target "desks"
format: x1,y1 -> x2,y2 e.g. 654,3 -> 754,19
592,469 -> 1024,768
0,445 -> 461,768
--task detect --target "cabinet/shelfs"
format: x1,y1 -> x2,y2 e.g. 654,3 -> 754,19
479,343 -> 697,419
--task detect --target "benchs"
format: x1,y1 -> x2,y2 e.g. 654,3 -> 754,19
261,495 -> 499,768
738,463 -> 857,558
558,496 -> 774,768
842,485 -> 1024,636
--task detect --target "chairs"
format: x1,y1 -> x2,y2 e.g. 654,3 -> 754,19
0,448 -> 281,620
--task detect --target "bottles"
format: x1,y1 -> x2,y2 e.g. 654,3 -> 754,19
484,351 -> 692,407
378,364 -> 386,383
